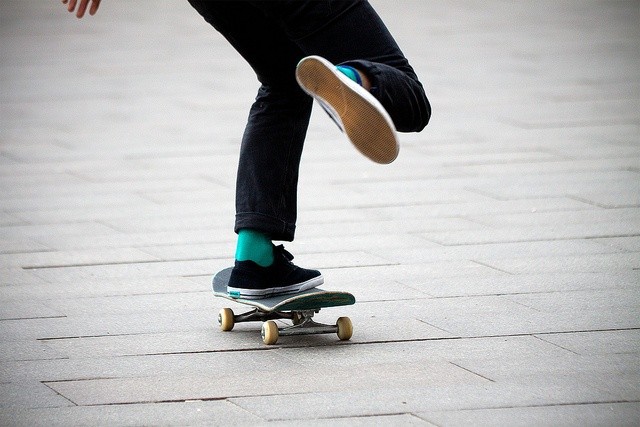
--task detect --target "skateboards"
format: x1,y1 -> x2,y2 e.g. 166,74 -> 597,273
212,266 -> 355,344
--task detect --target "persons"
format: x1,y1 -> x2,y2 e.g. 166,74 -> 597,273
61,0 -> 432,300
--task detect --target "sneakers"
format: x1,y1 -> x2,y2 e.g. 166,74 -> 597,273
294,55 -> 400,165
229,245 -> 322,298
